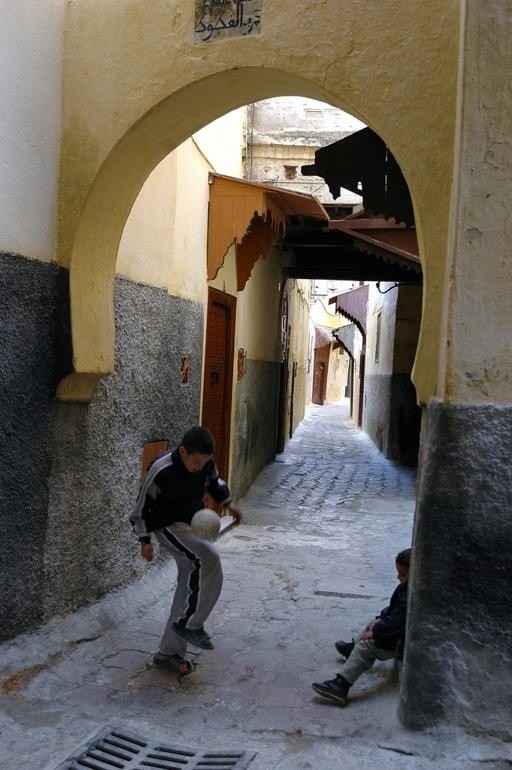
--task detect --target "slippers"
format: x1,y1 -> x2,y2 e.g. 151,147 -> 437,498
152,651 -> 192,676
169,621 -> 216,651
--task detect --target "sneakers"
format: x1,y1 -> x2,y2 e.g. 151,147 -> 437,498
333,639 -> 356,659
310,672 -> 351,704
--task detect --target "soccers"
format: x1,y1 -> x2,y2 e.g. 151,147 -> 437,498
192,509 -> 220,539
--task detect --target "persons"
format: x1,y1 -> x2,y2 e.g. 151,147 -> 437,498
127,423 -> 243,679
310,545 -> 413,708
